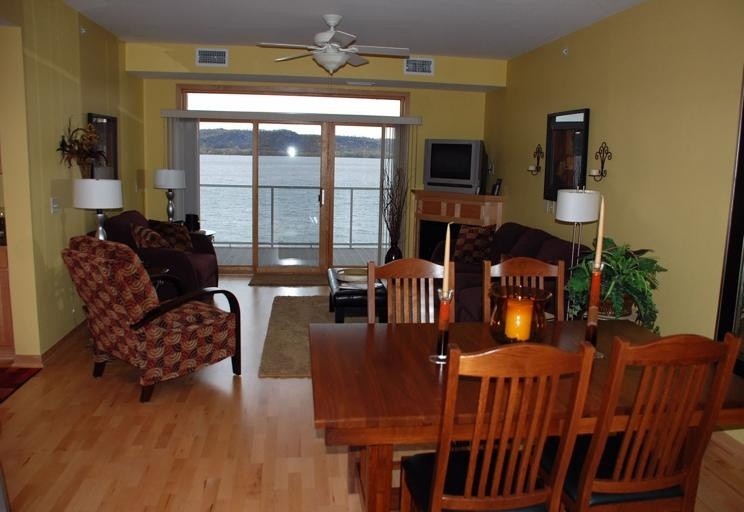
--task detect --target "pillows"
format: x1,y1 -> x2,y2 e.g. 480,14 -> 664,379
128,220 -> 172,253
452,221 -> 498,265
160,223 -> 194,250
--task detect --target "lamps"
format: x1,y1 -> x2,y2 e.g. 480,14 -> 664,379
311,51 -> 353,77
153,168 -> 187,224
554,185 -> 601,321
71,178 -> 125,242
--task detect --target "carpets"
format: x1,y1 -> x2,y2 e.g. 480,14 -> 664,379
0,365 -> 44,407
257,294 -> 380,379
247,272 -> 332,286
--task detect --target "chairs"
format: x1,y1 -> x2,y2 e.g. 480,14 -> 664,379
537,331 -> 743,512
481,257 -> 566,323
367,260 -> 456,327
398,337 -> 597,512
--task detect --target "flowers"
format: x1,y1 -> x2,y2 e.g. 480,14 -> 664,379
55,112 -> 109,168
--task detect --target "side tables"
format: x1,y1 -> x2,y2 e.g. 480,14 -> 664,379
189,228 -> 216,244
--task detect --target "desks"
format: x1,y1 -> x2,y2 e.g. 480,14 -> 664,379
310,317 -> 744,512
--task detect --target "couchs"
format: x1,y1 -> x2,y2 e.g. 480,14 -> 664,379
86,210 -> 219,302
444,221 -> 593,322
60,234 -> 242,403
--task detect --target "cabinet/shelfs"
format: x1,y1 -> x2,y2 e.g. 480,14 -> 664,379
409,188 -> 504,274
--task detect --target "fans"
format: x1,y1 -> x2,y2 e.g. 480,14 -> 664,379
254,13 -> 411,68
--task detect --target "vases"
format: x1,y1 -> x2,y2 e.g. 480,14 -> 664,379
76,158 -> 95,178
383,241 -> 404,289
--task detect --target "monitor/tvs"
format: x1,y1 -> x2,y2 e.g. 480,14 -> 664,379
423,138 -> 487,194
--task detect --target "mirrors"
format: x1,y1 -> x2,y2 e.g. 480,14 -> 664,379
543,107 -> 590,200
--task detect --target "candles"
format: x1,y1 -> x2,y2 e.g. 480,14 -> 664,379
528,164 -> 537,171
593,195 -> 604,271
504,294 -> 534,342
442,220 -> 451,295
588,170 -> 600,177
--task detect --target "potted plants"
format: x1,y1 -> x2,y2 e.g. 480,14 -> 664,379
563,237 -> 669,338
